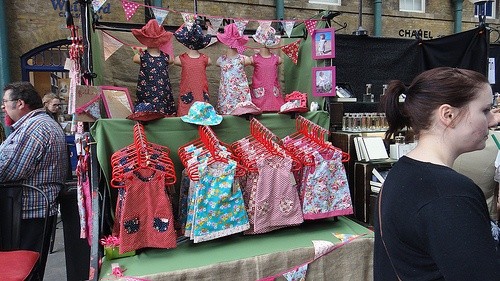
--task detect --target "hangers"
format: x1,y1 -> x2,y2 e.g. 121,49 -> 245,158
178,117 -> 353,182
68,25 -> 81,62
109,124 -> 176,188
80,134 -> 89,162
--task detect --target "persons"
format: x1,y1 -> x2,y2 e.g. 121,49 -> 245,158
172,22 -> 211,115
248,24 -> 284,112
373,68 -> 500,281
42,93 -> 61,120
318,34 -> 326,55
316,72 -> 329,91
0,82 -> 68,281
133,19 -> 177,115
216,23 -> 251,113
452,106 -> 500,214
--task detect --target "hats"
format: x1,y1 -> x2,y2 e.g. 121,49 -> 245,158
173,23 -> 212,50
319,72 -> 325,75
215,23 -> 248,46
230,101 -> 262,115
126,101 -> 165,122
180,101 -> 223,126
278,100 -> 308,114
132,18 -> 173,47
252,23 -> 281,46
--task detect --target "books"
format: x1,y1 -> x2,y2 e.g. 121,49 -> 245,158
354,137 -> 388,161
332,97 -> 357,102
370,168 -> 385,193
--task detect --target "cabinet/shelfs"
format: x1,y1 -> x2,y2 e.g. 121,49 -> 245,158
84,1 -> 375,281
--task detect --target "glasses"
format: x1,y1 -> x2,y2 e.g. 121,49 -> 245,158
1,98 -> 19,105
47,103 -> 62,107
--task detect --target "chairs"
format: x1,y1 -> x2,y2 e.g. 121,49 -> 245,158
0,184 -> 50,281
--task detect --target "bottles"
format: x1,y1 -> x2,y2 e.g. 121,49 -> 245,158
342,113 -> 388,131
